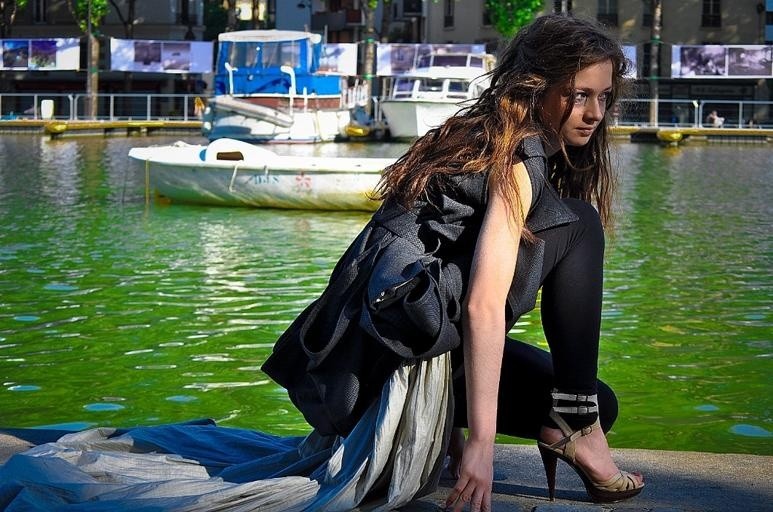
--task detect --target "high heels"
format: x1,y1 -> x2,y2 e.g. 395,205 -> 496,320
540,386 -> 644,504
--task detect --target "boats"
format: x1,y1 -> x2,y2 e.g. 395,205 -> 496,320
201,30 -> 368,142
378,51 -> 498,148
128,137 -> 402,213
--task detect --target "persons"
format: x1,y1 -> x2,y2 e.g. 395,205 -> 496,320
259,14 -> 646,511
